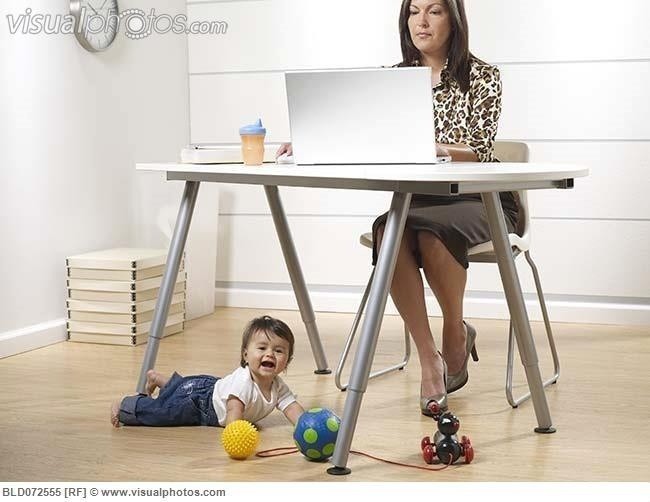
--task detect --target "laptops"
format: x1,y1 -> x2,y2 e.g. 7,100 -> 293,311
284,66 -> 452,164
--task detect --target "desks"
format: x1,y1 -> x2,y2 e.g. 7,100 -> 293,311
133,160 -> 588,477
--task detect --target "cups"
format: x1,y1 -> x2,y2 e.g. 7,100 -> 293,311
238,117 -> 267,165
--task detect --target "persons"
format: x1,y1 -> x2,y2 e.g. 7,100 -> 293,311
276,0 -> 521,418
220,418 -> 261,460
110,315 -> 304,429
293,407 -> 341,462
421,405 -> 474,465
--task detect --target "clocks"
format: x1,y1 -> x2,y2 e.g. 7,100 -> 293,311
69,0 -> 120,54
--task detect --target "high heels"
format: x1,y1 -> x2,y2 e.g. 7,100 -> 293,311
419,349 -> 449,419
447,319 -> 480,394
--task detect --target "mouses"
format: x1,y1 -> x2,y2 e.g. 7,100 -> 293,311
278,152 -> 294,165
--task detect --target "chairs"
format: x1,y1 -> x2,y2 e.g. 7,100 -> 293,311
334,140 -> 561,410
